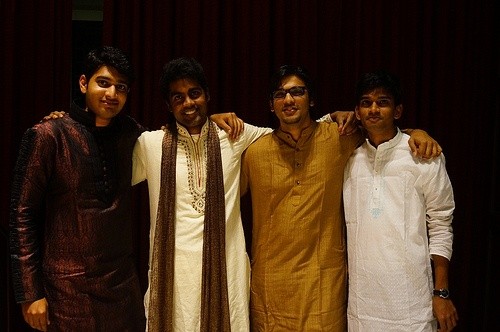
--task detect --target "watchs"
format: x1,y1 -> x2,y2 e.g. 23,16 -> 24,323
433,289 -> 449,298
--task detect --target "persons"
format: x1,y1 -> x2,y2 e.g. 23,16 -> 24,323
8,46 -> 244,332
160,64 -> 442,332
342,72 -> 459,332
43,57 -> 359,332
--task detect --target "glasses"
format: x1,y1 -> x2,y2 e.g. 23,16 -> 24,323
272,86 -> 308,98
